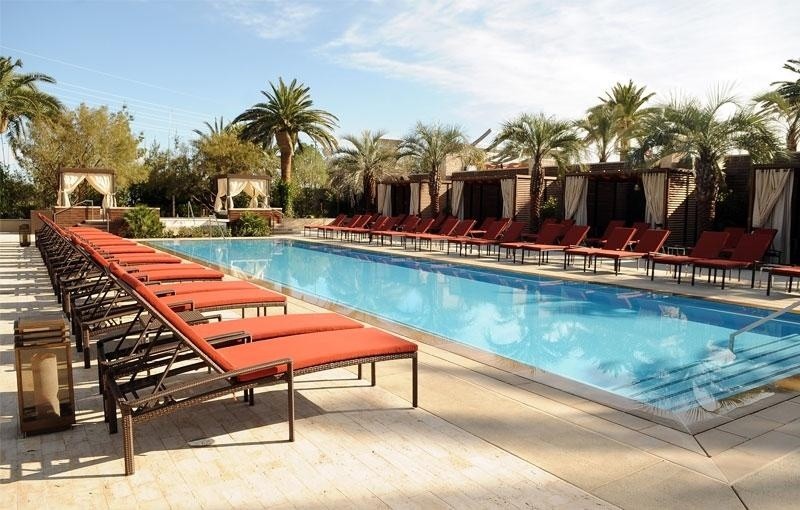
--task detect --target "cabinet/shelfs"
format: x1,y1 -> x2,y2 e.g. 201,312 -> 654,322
19,224 -> 32,247
14,315 -> 76,438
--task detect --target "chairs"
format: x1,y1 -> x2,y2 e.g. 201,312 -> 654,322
498,220 -> 590,267
563,220 -> 670,281
406,218 -> 526,265
648,227 -> 799,297
35,212 -> 224,285
304,213 -> 435,251
63,261 -> 288,368
99,261 -> 419,474
